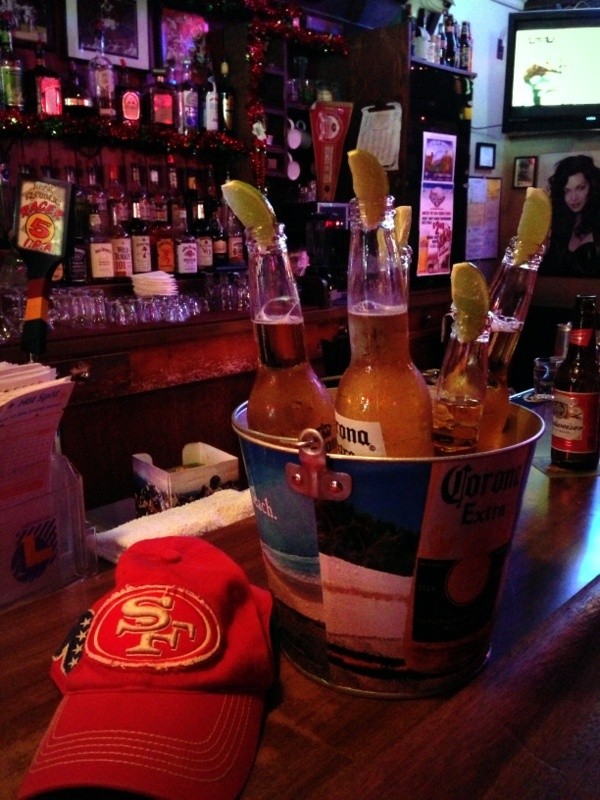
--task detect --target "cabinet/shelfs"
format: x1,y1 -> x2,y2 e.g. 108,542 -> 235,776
0,0 -> 477,510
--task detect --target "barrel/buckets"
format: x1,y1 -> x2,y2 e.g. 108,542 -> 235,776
230,375 -> 546,698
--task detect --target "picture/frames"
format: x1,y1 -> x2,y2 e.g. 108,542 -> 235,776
475,142 -> 496,169
65,0 -> 150,74
513,155 -> 538,189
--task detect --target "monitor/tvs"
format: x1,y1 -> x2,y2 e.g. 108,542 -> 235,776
503,8 -> 600,134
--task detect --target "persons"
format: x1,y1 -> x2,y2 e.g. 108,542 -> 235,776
537,155 -> 600,279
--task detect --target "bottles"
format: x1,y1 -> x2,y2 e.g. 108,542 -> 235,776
0,20 -> 600,481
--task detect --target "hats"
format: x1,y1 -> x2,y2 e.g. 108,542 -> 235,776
18,535 -> 273,800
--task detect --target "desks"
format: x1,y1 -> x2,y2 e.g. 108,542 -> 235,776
0,402 -> 600,800
